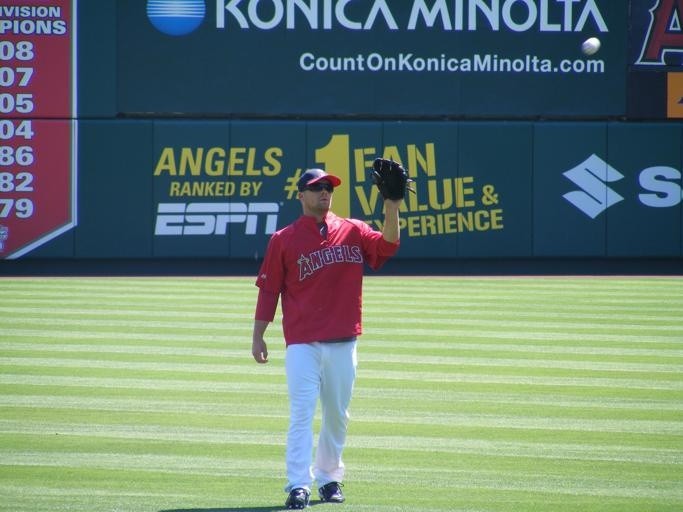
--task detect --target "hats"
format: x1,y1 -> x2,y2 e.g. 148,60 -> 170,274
296,168 -> 343,192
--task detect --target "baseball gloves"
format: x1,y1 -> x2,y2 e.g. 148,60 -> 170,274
372,157 -> 407,200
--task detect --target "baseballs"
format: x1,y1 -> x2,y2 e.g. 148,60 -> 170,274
583,37 -> 600,56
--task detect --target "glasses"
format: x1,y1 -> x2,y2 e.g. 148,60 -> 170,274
300,182 -> 334,192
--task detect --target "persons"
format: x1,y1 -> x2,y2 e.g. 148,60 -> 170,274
251,157 -> 410,512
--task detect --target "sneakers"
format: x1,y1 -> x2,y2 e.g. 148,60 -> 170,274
319,482 -> 346,503
285,488 -> 310,510
